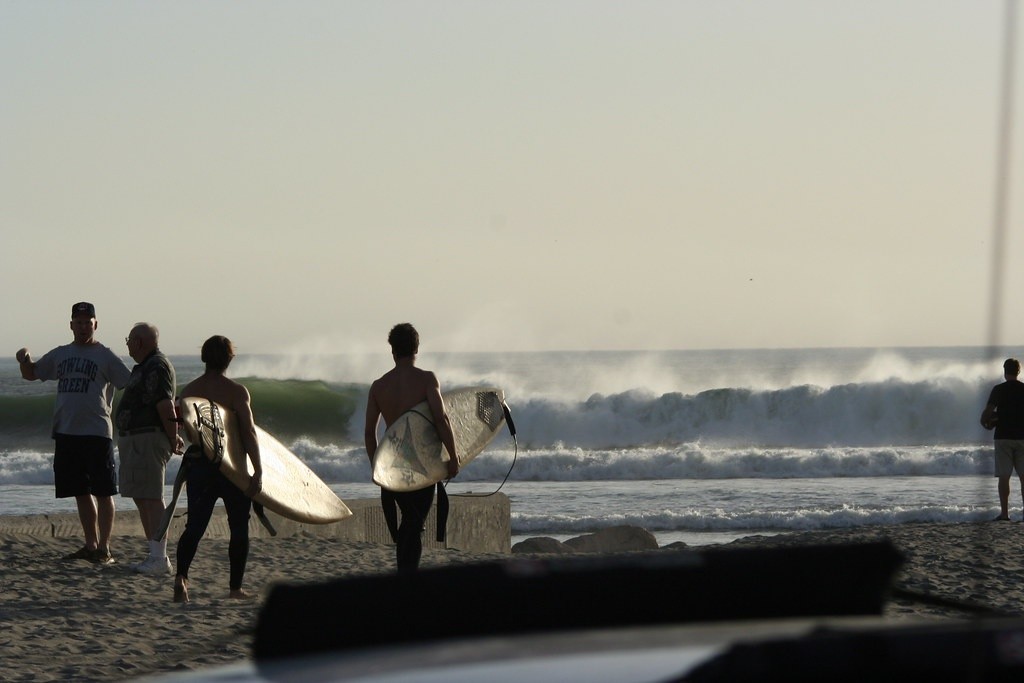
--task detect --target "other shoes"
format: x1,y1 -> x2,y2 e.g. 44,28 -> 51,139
992,514 -> 1010,522
173,576 -> 189,603
230,588 -> 259,600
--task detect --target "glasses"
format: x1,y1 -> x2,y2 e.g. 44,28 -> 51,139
125,337 -> 131,342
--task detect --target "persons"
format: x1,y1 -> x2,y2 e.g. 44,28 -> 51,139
16,302 -> 460,602
980,358 -> 1024,522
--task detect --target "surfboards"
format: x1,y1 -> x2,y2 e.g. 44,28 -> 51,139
178,396 -> 355,525
369,386 -> 511,492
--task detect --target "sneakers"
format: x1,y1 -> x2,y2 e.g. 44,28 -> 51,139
68,545 -> 97,559
135,555 -> 173,577
89,548 -> 111,564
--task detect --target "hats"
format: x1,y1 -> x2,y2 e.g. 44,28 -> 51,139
71,302 -> 96,319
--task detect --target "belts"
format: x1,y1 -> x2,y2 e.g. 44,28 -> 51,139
118,428 -> 155,438
